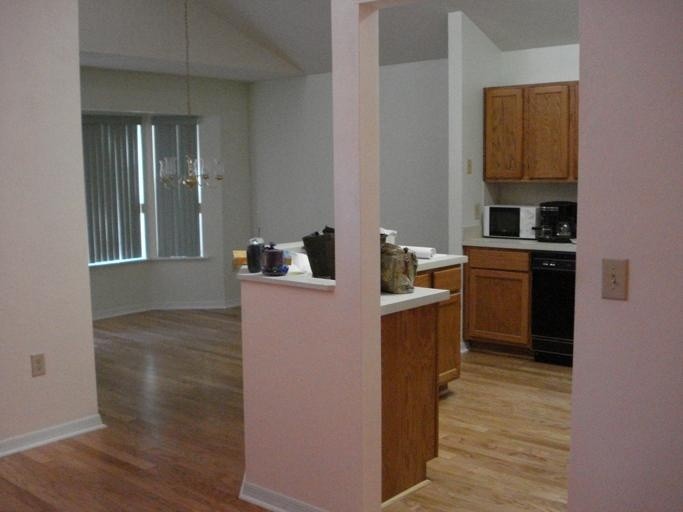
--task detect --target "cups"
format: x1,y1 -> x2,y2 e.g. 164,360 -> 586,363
284,255 -> 293,266
263,250 -> 283,267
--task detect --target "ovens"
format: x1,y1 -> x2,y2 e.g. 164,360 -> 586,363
530,250 -> 575,367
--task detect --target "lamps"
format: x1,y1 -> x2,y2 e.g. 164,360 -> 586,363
154,0 -> 229,192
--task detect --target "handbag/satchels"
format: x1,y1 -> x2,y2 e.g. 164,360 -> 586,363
379,243 -> 417,294
301,227 -> 334,279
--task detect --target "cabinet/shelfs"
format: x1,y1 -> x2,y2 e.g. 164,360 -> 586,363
481,81 -> 579,182
464,245 -> 533,350
231,236 -> 470,512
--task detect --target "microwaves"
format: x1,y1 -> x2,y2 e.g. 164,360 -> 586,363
482,202 -> 539,240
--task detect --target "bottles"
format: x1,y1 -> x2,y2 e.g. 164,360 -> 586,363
246,245 -> 262,273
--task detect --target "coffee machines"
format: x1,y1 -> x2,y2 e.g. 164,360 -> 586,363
539,201 -> 575,243
